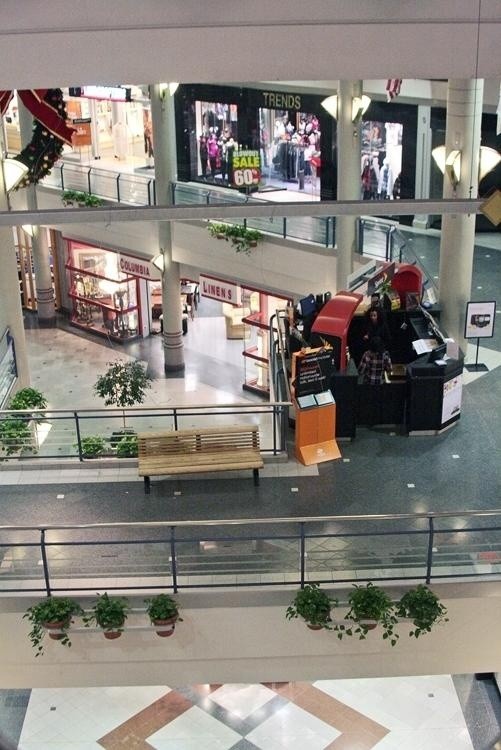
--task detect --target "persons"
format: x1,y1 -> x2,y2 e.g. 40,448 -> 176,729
200,125 -> 235,185
359,308 -> 392,386
293,116 -> 321,176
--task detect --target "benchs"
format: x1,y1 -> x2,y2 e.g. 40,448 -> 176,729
137,426 -> 264,494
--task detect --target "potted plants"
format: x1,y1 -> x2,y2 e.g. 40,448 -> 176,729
59,189 -> 75,207
89,592 -> 129,639
226,224 -> 246,244
22,598 -> 84,657
344,581 -> 399,647
286,582 -> 338,630
207,223 -> 226,239
144,593 -> 179,636
236,230 -> 261,256
92,357 -> 156,459
86,195 -> 104,208
73,192 -> 87,208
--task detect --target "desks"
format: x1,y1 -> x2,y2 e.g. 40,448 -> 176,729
159,313 -> 188,335
152,295 -> 188,319
180,283 -> 197,320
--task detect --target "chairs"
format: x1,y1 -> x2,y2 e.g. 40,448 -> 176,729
221,303 -> 252,339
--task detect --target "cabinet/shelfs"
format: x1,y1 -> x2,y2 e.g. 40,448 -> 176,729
64,256 -> 143,344
